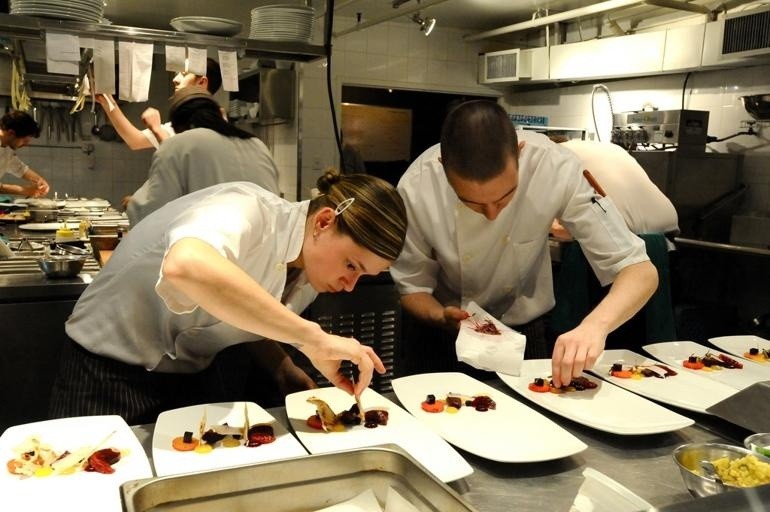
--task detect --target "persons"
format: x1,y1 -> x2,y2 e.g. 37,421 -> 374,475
89,57 -> 230,153
386,97 -> 662,387
120,85 -> 280,231
551,139 -> 685,355
45,169 -> 409,419
0,111 -> 50,199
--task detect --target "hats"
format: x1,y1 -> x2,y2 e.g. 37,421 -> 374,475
168,85 -> 215,117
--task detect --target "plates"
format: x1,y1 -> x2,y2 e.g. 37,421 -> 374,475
497,358 -> 696,435
1,195 -> 131,232
588,334 -> 770,417
152,400 -> 311,477
168,16 -> 245,37
248,4 -> 316,44
390,372 -> 589,464
9,0 -> 113,26
226,98 -> 247,117
1,413 -> 154,501
284,383 -> 477,485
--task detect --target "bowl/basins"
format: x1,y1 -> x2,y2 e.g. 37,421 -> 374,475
36,245 -> 88,280
672,433 -> 770,500
738,93 -> 770,122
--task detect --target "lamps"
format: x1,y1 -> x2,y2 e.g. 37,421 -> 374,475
406,10 -> 438,36
